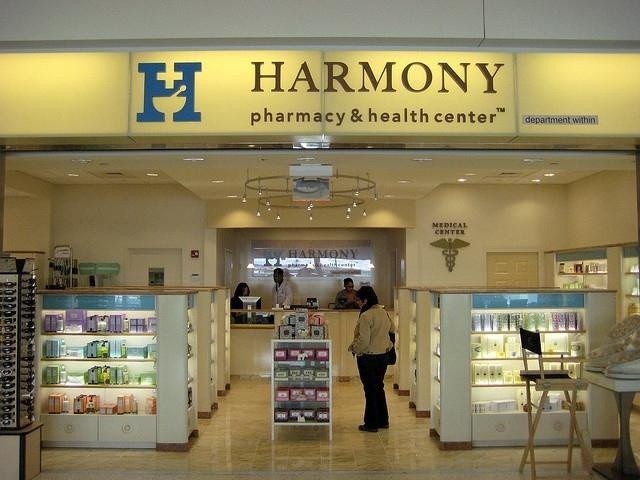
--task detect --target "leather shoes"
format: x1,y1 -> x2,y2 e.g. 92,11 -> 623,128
359,423 -> 389,432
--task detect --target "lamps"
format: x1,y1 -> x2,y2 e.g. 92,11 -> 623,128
237,161 -> 383,231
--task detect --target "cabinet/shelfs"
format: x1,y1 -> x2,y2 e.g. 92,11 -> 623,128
558,271 -> 640,299
390,284 -> 410,399
408,288 -> 433,420
212,284 -> 233,398
268,336 -> 335,440
192,285 -> 218,425
35,288 -> 201,455
427,285 -> 623,452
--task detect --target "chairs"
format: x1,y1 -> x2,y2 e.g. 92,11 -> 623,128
513,327 -> 588,480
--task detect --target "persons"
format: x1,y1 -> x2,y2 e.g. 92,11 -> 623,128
230,282 -> 250,322
346,284 -> 398,432
270,268 -> 293,311
334,278 -> 359,308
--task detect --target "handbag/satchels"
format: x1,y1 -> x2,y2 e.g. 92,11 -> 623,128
386,331 -> 396,364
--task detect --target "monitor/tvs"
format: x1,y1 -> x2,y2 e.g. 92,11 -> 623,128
238,296 -> 261,324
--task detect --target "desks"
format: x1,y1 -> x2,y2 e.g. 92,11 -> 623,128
578,357 -> 640,480
